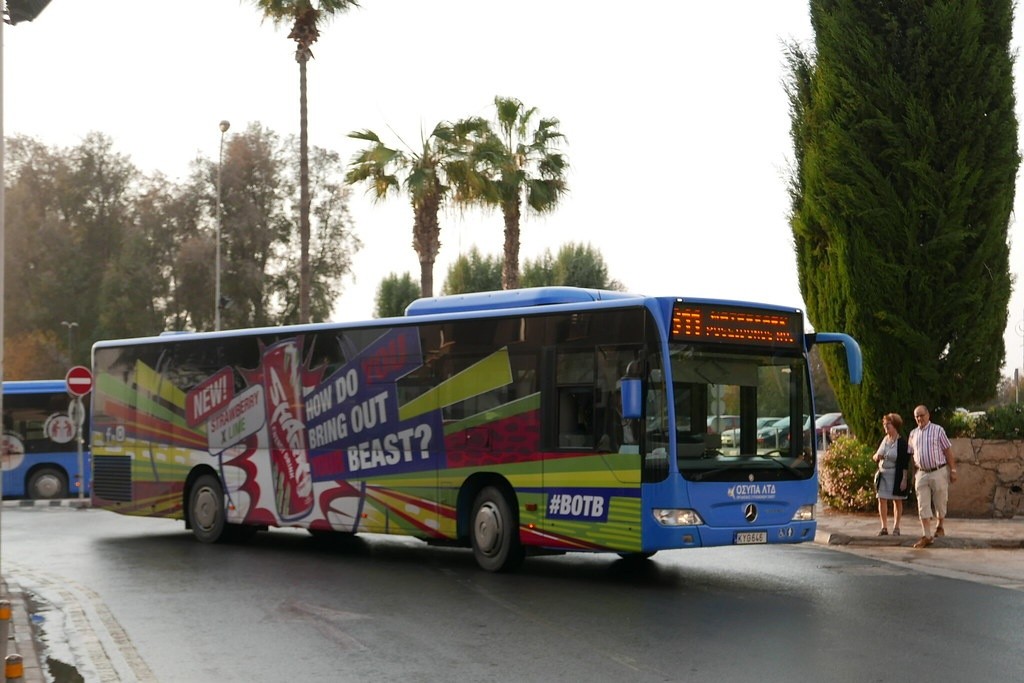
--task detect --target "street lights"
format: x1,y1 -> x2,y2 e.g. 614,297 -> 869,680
215,119 -> 234,328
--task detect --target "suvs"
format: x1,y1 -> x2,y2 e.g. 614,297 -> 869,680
646,416 -> 690,435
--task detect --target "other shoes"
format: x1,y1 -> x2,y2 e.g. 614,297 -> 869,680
878,528 -> 888,536
892,528 -> 900,536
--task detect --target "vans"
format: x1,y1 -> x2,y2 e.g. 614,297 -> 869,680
707,416 -> 739,437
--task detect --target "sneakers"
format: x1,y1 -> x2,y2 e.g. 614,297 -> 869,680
935,528 -> 945,538
913,536 -> 934,549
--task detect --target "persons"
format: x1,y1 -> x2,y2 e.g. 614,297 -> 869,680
595,391 -> 646,468
873,413 -> 911,537
908,405 -> 957,550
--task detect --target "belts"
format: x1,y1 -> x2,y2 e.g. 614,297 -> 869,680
918,463 -> 948,473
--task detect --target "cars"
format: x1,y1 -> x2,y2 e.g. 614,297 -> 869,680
788,413 -> 847,449
830,425 -> 853,441
721,417 -> 784,448
757,414 -> 810,449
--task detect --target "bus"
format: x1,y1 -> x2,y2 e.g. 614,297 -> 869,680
89,282 -> 863,574
0,381 -> 93,500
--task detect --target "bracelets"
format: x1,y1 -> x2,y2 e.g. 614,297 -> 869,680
901,475 -> 908,481
952,469 -> 957,473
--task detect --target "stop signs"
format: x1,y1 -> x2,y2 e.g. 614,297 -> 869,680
65,366 -> 92,397
